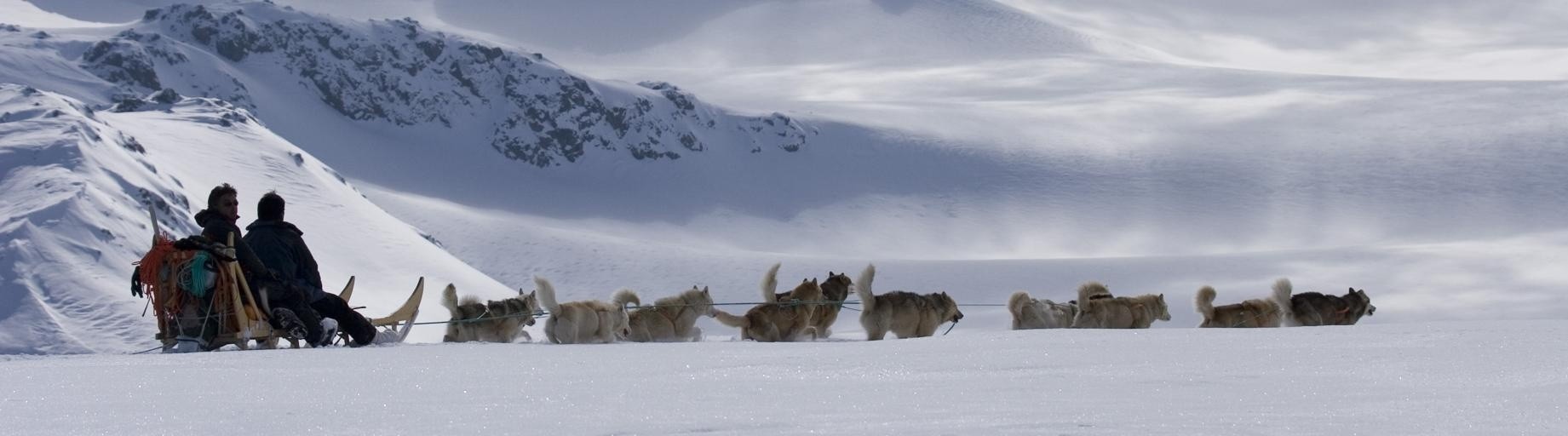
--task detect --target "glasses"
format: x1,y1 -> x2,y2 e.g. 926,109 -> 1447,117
215,201 -> 239,207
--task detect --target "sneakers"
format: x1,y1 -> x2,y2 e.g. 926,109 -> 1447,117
312,317 -> 337,348
370,329 -> 398,344
273,308 -> 308,339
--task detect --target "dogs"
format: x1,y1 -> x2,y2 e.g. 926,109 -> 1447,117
1193,284 -> 1286,329
1272,277 -> 1377,329
761,261 -> 857,340
530,274 -> 632,344
439,281 -> 544,345
1071,284 -> 1174,330
856,263 -> 964,341
613,284 -> 719,344
1007,289 -> 1078,328
716,277 -> 824,344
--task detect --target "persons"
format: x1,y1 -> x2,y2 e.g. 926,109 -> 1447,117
244,191 -> 398,345
196,184 -> 337,346
